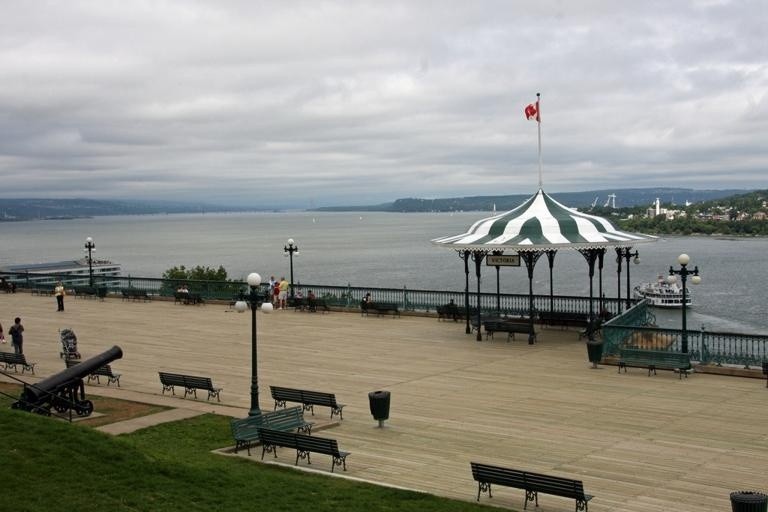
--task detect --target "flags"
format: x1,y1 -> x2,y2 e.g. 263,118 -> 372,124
525,99 -> 542,122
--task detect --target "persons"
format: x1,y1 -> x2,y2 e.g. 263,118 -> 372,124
0,323 -> 5,344
447,298 -> 464,323
178,285 -> 185,304
6,316 -> 25,355
182,284 -> 191,304
267,275 -> 316,312
361,293 -> 372,303
54,280 -> 65,312
586,306 -> 613,342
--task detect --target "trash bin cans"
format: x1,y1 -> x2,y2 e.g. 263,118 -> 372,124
730,491 -> 768,512
587,341 -> 603,368
368,391 -> 390,426
762,359 -> 768,387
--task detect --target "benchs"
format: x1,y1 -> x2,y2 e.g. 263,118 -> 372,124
158,371 -> 224,404
229,294 -> 268,310
120,288 -> 152,303
0,351 -> 122,389
74,286 -> 106,300
229,385 -> 350,474
293,298 -> 331,314
360,301 -> 401,319
174,292 -> 204,306
32,285 -> 57,297
437,302 -> 693,380
469,461 -> 595,511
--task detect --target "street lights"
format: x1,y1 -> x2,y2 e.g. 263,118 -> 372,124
281,238 -> 300,298
235,271 -> 274,418
667,253 -> 703,353
82,235 -> 97,288
615,247 -> 641,310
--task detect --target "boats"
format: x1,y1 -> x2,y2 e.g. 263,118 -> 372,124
633,275 -> 693,308
0,256 -> 123,291
